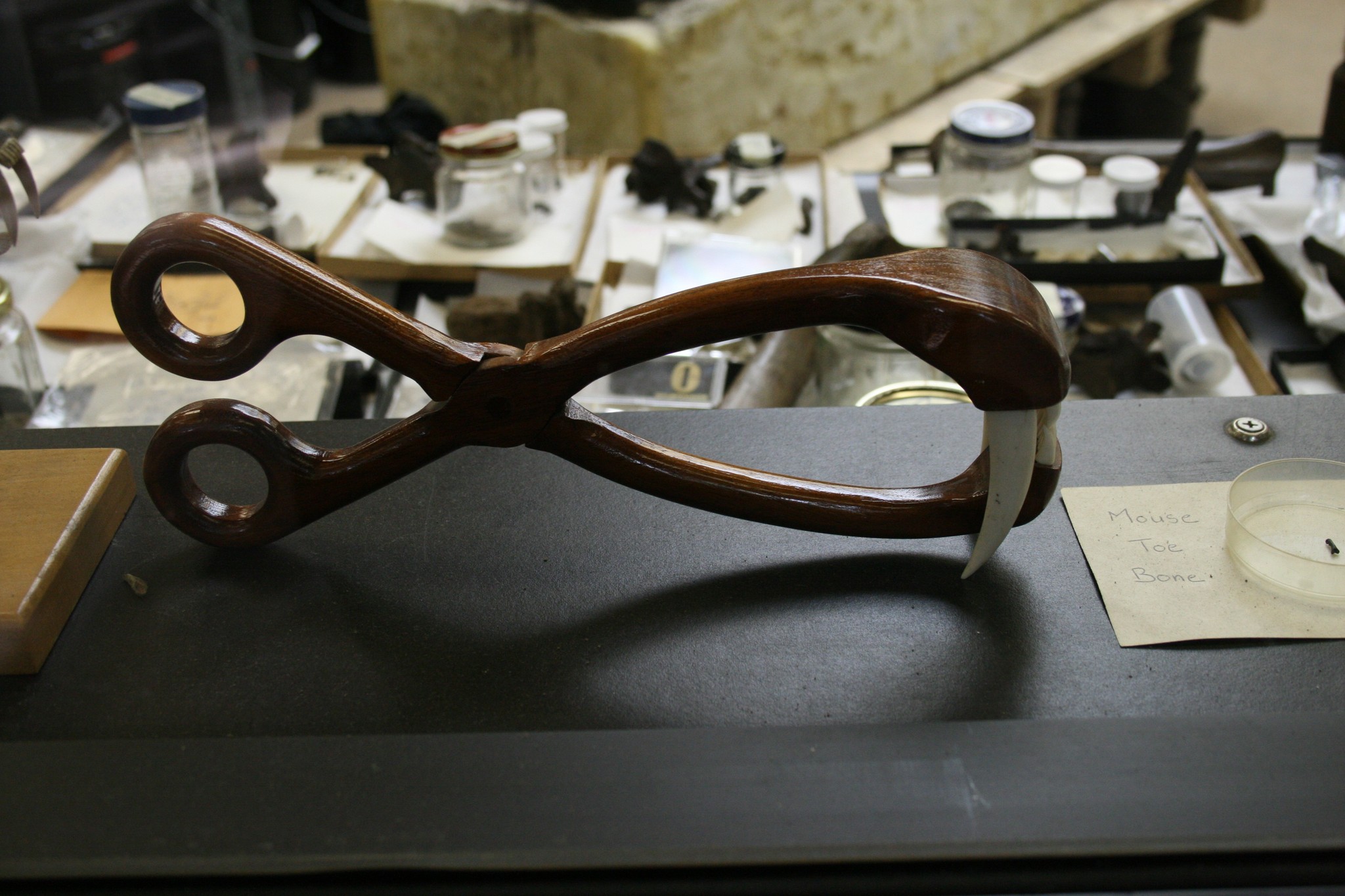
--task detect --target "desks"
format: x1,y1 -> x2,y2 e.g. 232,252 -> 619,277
0,147 -> 1345,896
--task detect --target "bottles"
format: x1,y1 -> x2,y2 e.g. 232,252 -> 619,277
811,321 -> 974,406
435,106 -> 571,249
721,131 -> 789,223
936,95 -> 1158,220
0,276 -> 49,433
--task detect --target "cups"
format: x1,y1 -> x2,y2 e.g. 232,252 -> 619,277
1147,285 -> 1235,395
121,77 -> 222,216
